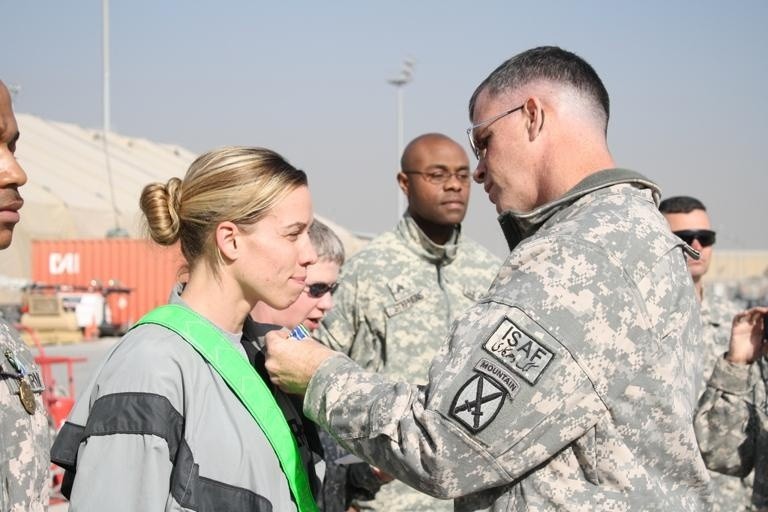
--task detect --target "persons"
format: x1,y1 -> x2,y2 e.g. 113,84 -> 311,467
0,81 -> 57,512
694,305 -> 767,512
251,219 -> 344,512
46,145 -> 326,512
311,131 -> 504,512
262,47 -> 714,512
658,194 -> 751,512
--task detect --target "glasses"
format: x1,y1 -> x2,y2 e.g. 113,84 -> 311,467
405,169 -> 475,185
672,229 -> 715,247
306,281 -> 339,297
466,104 -> 524,160
